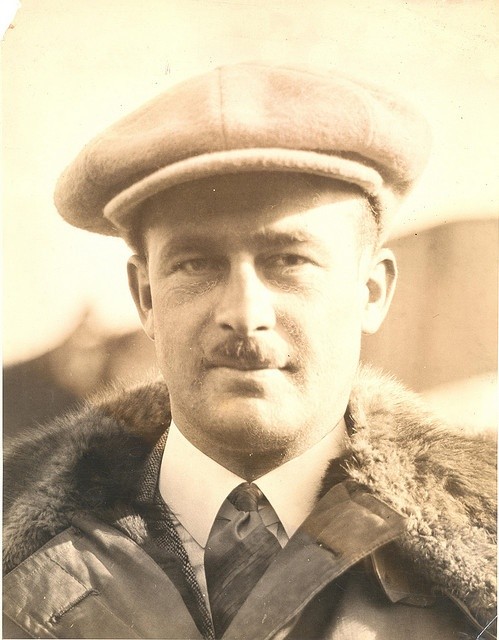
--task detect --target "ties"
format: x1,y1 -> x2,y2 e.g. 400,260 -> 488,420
204,483 -> 282,640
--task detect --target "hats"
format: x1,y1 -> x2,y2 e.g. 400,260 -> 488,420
54,59 -> 433,254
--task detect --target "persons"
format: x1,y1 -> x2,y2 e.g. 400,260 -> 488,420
4,59 -> 497,639
